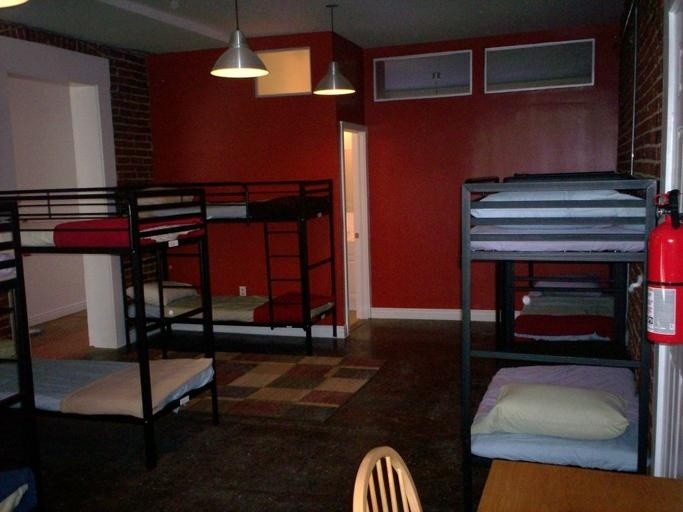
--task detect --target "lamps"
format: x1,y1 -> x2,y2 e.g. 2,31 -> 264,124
312,5 -> 356,95
210,0 -> 270,78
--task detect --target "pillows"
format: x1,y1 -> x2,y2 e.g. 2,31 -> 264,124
136,187 -> 194,205
126,281 -> 199,306
0,339 -> 17,359
529,274 -> 602,297
471,383 -> 630,440
470,191 -> 632,229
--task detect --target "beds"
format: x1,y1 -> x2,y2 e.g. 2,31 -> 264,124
0,201 -> 45,512
0,188 -> 220,471
117,180 -> 337,356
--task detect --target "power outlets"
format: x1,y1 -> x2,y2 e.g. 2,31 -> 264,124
240,286 -> 246,297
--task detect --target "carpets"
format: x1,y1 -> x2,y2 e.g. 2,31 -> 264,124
121,348 -> 387,423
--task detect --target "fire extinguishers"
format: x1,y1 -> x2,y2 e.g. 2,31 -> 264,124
645,189 -> 683,345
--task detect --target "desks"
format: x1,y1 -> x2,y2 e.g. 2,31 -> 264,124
477,460 -> 683,512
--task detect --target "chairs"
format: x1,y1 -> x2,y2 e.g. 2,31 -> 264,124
352,446 -> 423,512
